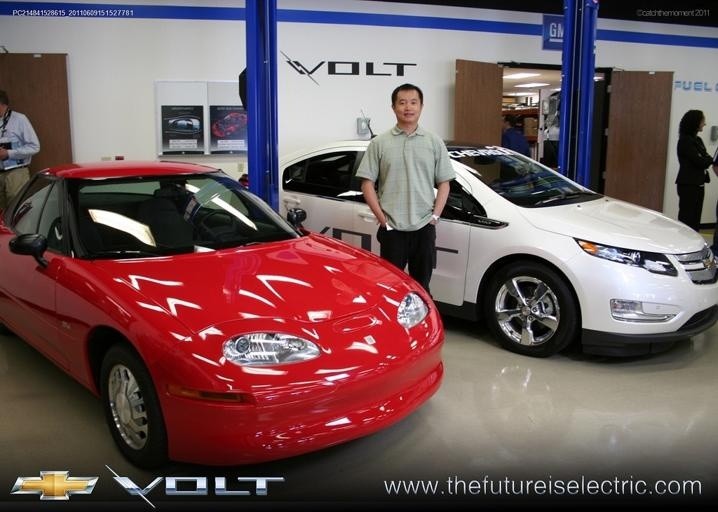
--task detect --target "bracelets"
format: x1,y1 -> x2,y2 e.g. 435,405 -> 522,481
432,214 -> 441,224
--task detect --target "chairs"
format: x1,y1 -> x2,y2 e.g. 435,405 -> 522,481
129,198 -> 196,252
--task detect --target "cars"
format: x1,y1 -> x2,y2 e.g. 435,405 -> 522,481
212,112 -> 248,137
239,139 -> 718,360
163,115 -> 202,134
0,158 -> 447,473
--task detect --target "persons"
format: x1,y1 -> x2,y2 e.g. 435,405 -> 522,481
674,109 -> 713,233
502,113 -> 530,157
353,83 -> 456,301
710,146 -> 717,257
0,87 -> 41,216
